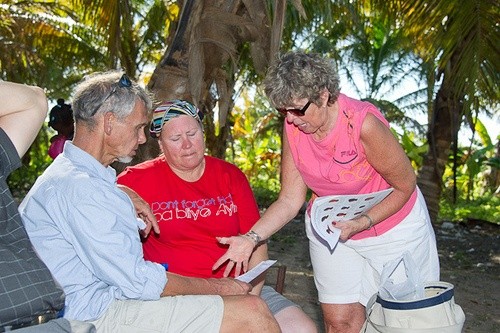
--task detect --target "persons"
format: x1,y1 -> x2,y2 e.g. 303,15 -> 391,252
0,78 -> 72,333
47,98 -> 74,160
18,68 -> 283,333
117,98 -> 318,333
212,47 -> 439,333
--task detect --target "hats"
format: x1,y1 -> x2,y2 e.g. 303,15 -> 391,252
149,100 -> 204,138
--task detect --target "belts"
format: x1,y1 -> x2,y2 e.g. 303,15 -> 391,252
0,311 -> 59,333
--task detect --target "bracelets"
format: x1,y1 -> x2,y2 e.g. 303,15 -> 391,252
361,214 -> 372,229
246,231 -> 261,245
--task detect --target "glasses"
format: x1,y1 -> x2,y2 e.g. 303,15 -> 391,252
275,98 -> 313,117
91,74 -> 131,116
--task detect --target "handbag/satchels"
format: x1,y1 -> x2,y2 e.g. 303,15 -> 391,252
360,281 -> 465,333
